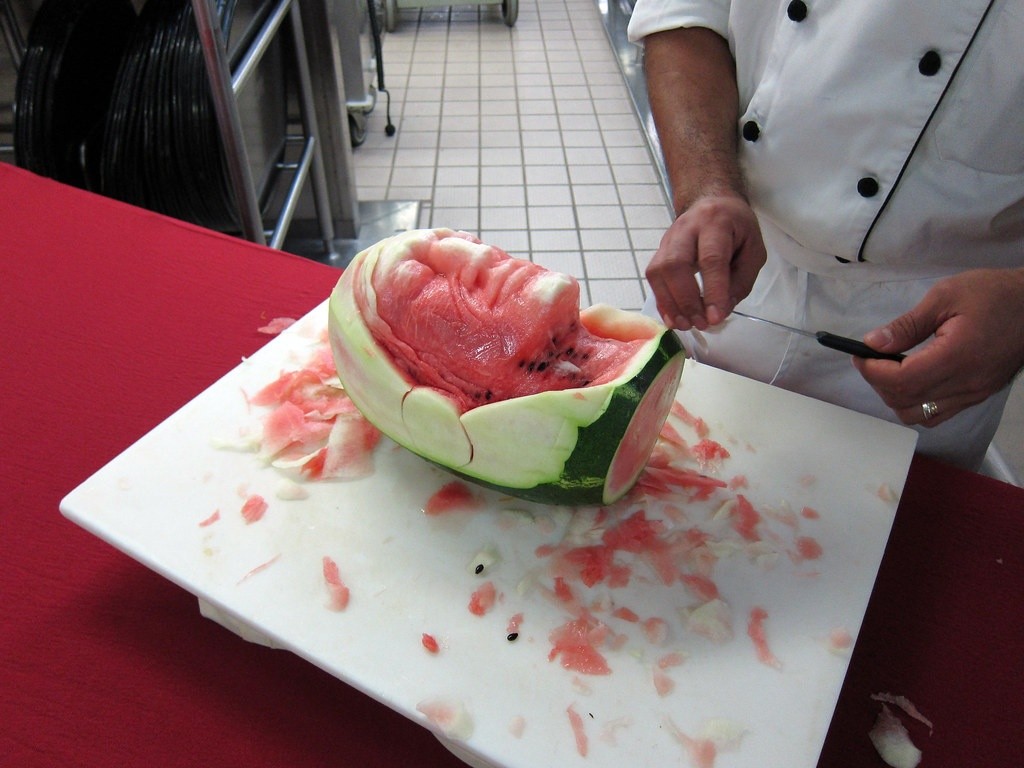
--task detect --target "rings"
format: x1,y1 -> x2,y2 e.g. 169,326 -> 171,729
922,400 -> 941,420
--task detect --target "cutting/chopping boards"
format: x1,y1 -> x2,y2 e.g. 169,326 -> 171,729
59,301 -> 920,768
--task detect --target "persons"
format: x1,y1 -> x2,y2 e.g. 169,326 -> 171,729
353,221 -> 655,421
626,0 -> 1024,486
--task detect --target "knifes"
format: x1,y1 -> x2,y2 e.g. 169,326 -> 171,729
698,296 -> 909,364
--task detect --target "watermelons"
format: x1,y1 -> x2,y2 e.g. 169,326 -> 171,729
328,228 -> 688,506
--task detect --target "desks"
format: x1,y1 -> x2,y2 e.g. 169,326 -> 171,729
1,161 -> 1024,768
